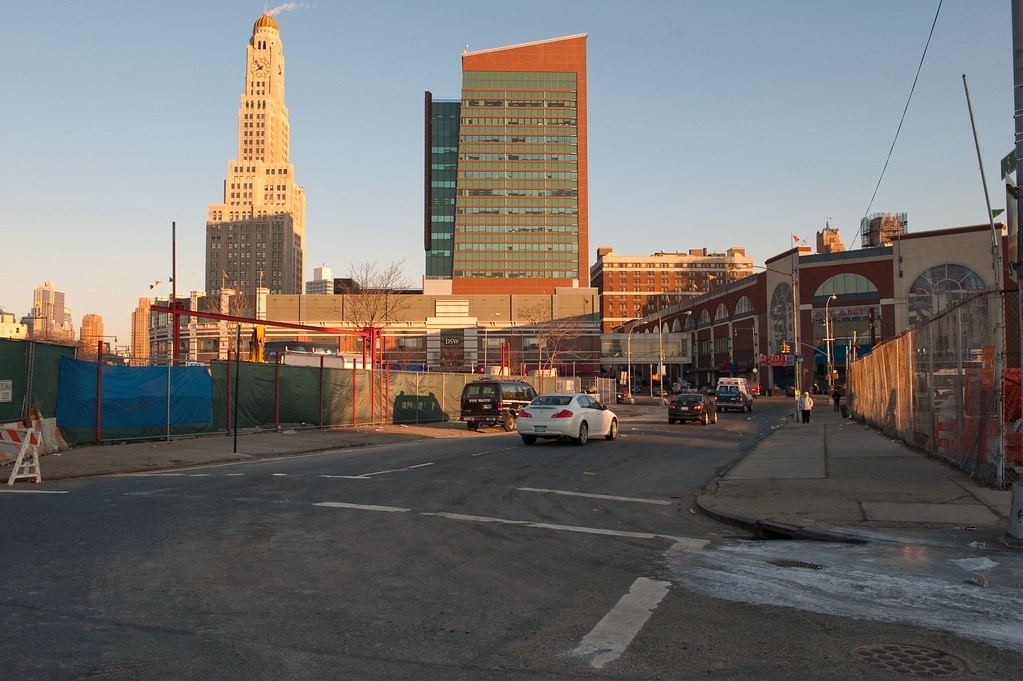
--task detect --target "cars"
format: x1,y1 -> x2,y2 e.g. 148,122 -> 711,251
632,387 -> 641,394
714,392 -> 749,413
668,393 -> 720,424
586,387 -> 626,404
653,388 -> 667,398
515,393 -> 619,447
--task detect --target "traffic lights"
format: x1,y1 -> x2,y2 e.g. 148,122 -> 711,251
662,352 -> 667,362
780,344 -> 790,354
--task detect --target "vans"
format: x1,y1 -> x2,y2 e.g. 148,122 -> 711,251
458,380 -> 541,434
748,380 -> 760,396
715,384 -> 754,412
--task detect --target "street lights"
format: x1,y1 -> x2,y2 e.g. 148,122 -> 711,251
483,313 -> 500,374
626,321 -> 649,398
825,295 -> 836,392
659,311 -> 693,400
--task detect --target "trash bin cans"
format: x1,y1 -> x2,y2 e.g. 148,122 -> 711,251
840,404 -> 849,418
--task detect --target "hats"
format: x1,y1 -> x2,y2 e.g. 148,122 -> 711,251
804,392 -> 808,394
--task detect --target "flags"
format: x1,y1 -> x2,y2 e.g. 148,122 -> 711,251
792,234 -> 799,242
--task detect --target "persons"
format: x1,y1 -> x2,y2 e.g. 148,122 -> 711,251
832,389 -> 841,412
800,391 -> 814,424
